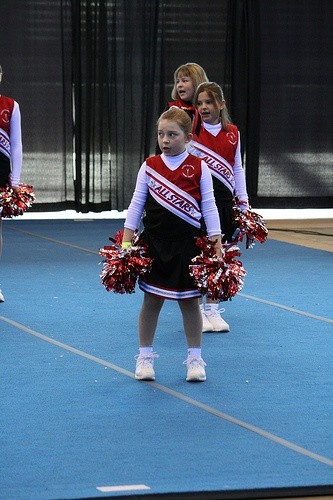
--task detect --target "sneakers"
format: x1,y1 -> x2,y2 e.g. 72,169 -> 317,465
183,355 -> 207,381
205,304 -> 230,332
0,289 -> 5,302
199,304 -> 214,332
134,352 -> 160,379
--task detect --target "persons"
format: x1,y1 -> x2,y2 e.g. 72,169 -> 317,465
121,107 -> 222,382
155,63 -> 210,155
185,82 -> 249,332
0,65 -> 22,301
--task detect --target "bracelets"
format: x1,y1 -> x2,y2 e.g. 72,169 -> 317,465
122,242 -> 132,248
